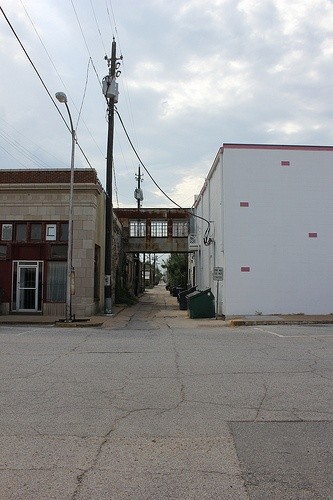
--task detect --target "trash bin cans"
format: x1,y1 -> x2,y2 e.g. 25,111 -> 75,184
185,287 -> 215,319
177,284 -> 198,310
166,284 -> 186,297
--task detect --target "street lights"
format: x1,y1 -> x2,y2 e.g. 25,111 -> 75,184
55,91 -> 77,323
